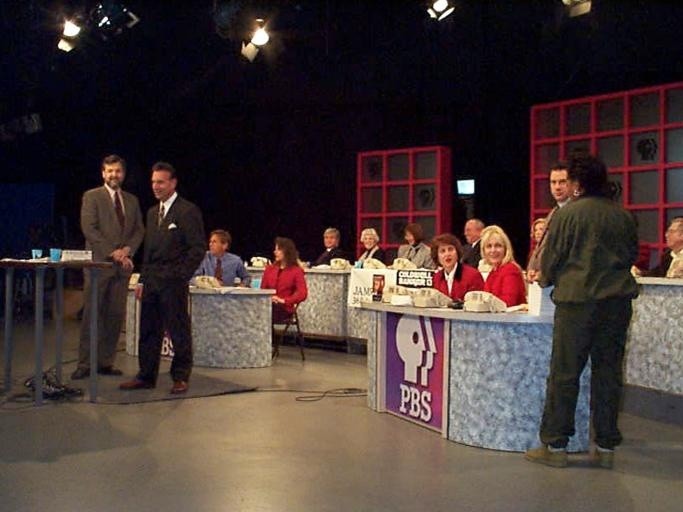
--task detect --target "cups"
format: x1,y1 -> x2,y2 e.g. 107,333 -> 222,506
253,279 -> 261,288
354,261 -> 362,269
50,248 -> 61,261
31,250 -> 42,259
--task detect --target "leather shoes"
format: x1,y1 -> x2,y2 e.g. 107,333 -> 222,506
24,376 -> 65,399
118,376 -> 156,390
72,364 -> 89,379
97,366 -> 123,376
171,380 -> 190,394
42,369 -> 82,395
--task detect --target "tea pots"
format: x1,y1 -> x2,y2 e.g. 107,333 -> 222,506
373,274 -> 385,301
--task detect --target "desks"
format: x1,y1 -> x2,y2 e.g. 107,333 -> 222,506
0,259 -> 113,406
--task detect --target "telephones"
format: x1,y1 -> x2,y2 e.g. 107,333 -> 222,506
464,291 -> 506,312
363,258 -> 385,269
330,259 -> 352,269
414,288 -> 451,307
250,257 -> 271,268
196,276 -> 220,288
382,286 -> 413,302
393,258 -> 416,269
478,259 -> 494,273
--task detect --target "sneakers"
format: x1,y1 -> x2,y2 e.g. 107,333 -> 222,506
523,443 -> 568,468
593,445 -> 615,469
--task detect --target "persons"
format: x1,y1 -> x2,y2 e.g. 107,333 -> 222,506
305,227 -> 354,268
529,218 -> 548,246
70,153 -> 147,382
259,235 -> 308,361
525,160 -> 572,285
430,234 -> 484,307
117,161 -> 207,394
355,227 -> 386,264
478,224 -> 528,313
627,215 -> 683,279
460,218 -> 486,269
191,228 -> 252,289
396,222 -> 437,271
521,154 -> 642,468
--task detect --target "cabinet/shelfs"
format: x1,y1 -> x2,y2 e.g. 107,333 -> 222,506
357,146 -> 452,266
528,82 -> 682,274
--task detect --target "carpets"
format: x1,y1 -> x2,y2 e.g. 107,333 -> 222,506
90,368 -> 257,404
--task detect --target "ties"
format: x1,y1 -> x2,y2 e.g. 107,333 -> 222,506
114,192 -> 125,226
160,205 -> 164,220
214,259 -> 222,280
